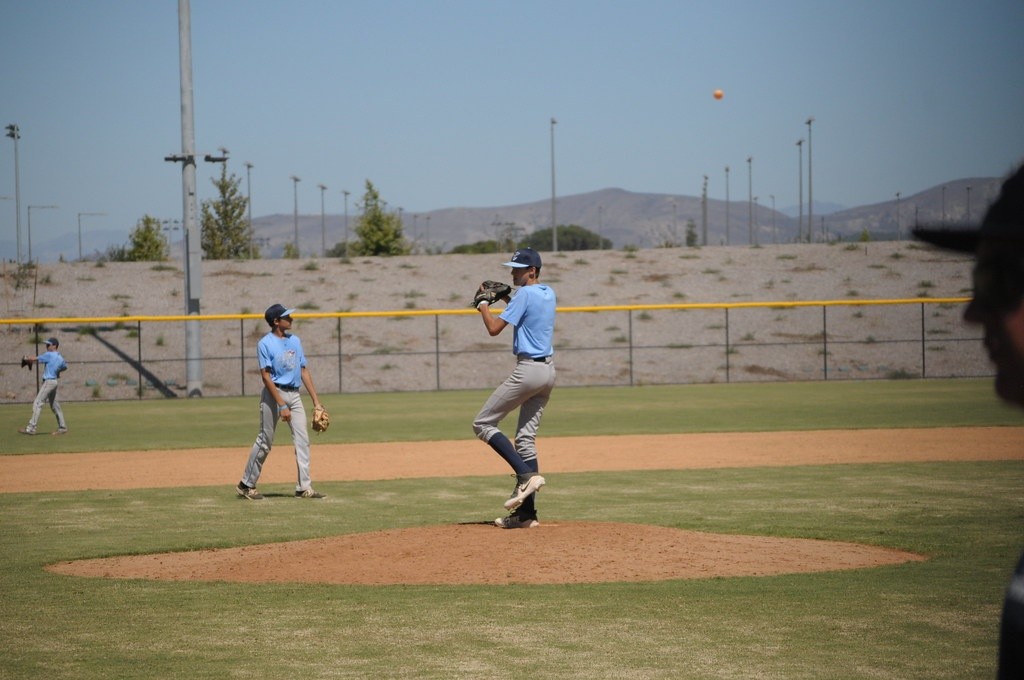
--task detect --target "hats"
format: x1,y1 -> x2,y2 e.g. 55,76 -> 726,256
42,338 -> 59,346
500,246 -> 541,268
264,304 -> 296,323
909,162 -> 1024,279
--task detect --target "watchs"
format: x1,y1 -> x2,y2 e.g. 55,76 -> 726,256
279,404 -> 287,410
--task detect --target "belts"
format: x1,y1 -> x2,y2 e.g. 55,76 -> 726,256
534,357 -> 546,362
274,383 -> 299,392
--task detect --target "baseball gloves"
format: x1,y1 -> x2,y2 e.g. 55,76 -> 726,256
21,355 -> 33,371
472,280 -> 512,312
310,404 -> 330,433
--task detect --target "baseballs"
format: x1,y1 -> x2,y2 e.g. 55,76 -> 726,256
712,89 -> 723,100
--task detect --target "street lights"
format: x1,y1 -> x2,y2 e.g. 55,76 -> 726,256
896,184 -> 971,241
804,116 -> 815,243
5,122 -> 22,278
549,116 -> 557,251
290,175 -> 300,257
796,138 -> 806,242
724,156 -> 776,246
245,161 -> 254,259
318,184 -> 327,254
342,190 -> 351,258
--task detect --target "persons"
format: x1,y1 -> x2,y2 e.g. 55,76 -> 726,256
911,164 -> 1024,680
236,304 -> 329,499
473,248 -> 557,528
18,336 -> 68,435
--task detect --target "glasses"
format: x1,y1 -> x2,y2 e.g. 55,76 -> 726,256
972,262 -> 1024,316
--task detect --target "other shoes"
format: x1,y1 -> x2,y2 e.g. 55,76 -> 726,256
17,428 -> 35,435
52,429 -> 65,436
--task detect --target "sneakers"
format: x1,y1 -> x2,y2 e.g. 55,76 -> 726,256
295,489 -> 327,498
494,508 -> 539,528
504,472 -> 545,510
236,481 -> 264,500
